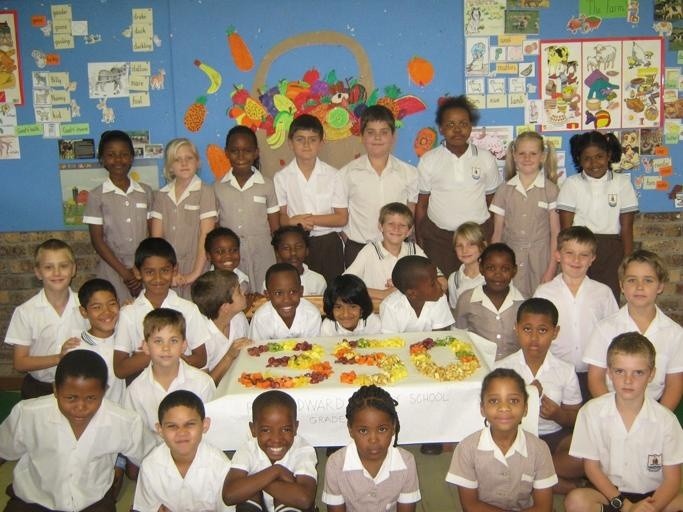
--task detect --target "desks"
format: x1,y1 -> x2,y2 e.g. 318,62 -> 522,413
243,294 -> 326,317
208,328 -> 492,453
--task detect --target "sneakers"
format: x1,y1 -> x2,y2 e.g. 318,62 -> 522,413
420,443 -> 443,455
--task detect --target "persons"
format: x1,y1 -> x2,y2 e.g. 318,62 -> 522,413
82,95 -> 638,307
1,225 -> 683,512
